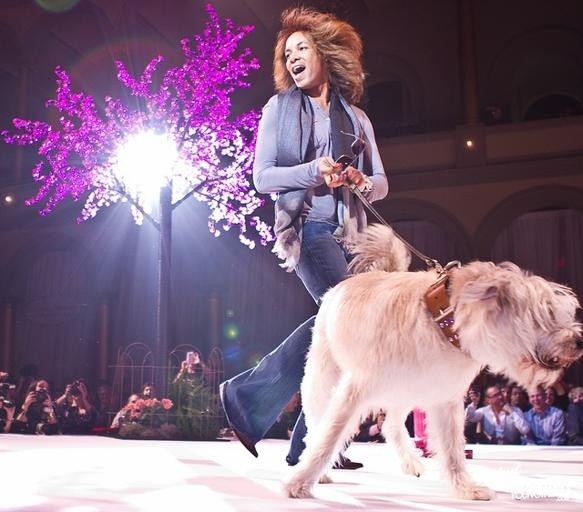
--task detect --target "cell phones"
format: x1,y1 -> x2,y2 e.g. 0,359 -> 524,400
187,352 -> 195,364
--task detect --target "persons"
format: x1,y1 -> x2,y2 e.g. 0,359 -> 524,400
463,380 -> 583,445
112,393 -> 140,427
220,6 -> 389,471
0,369 -> 112,433
171,351 -> 216,392
142,380 -> 156,397
265,392 -> 386,443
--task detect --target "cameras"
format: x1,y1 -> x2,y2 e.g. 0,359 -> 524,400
71,380 -> 80,396
34,389 -> 47,401
3,400 -> 15,408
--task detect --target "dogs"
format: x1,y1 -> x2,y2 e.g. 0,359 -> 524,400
283,223 -> 583,503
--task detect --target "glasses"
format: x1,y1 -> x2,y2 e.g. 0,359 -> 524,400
322,129 -> 367,174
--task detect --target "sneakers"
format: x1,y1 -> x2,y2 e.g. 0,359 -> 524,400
218,382 -> 259,459
287,454 -> 364,471
